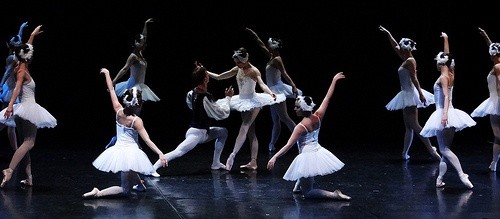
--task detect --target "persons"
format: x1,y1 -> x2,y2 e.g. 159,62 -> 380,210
266,72 -> 351,201
107,18 -> 160,149
471,28 -> 500,172
419,32 -> 477,188
83,68 -> 169,198
246,27 -> 304,154
0,25 -> 57,188
195,47 -> 286,171
152,67 -> 234,177
379,25 -> 441,161
0,22 -> 28,153
